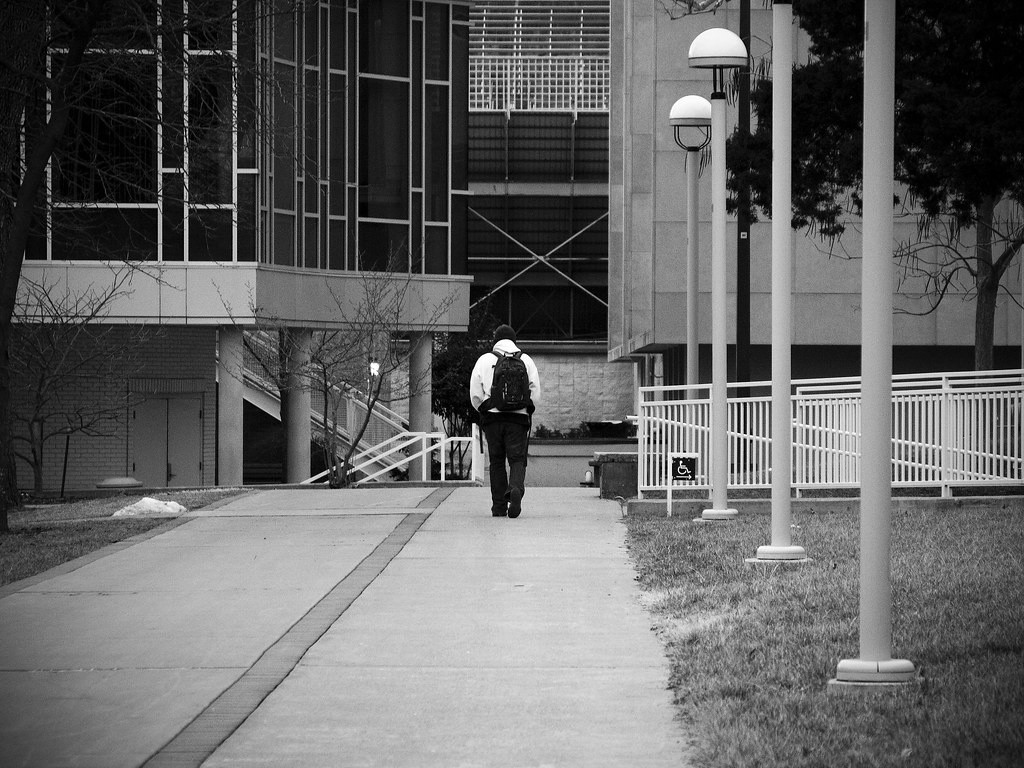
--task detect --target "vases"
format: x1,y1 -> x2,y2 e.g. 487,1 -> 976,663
587,422 -> 630,439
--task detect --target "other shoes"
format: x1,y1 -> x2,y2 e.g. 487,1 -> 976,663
492,509 -> 507,517
508,487 -> 522,518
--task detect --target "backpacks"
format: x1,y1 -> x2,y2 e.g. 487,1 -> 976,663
490,352 -> 532,411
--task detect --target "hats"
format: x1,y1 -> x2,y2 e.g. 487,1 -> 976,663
494,324 -> 517,343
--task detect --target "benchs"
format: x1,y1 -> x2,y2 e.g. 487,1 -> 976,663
589,452 -> 662,499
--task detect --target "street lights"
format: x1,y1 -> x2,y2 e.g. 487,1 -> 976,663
670,94 -> 712,453
687,27 -> 750,521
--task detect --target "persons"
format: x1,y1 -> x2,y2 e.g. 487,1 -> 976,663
469,325 -> 539,517
